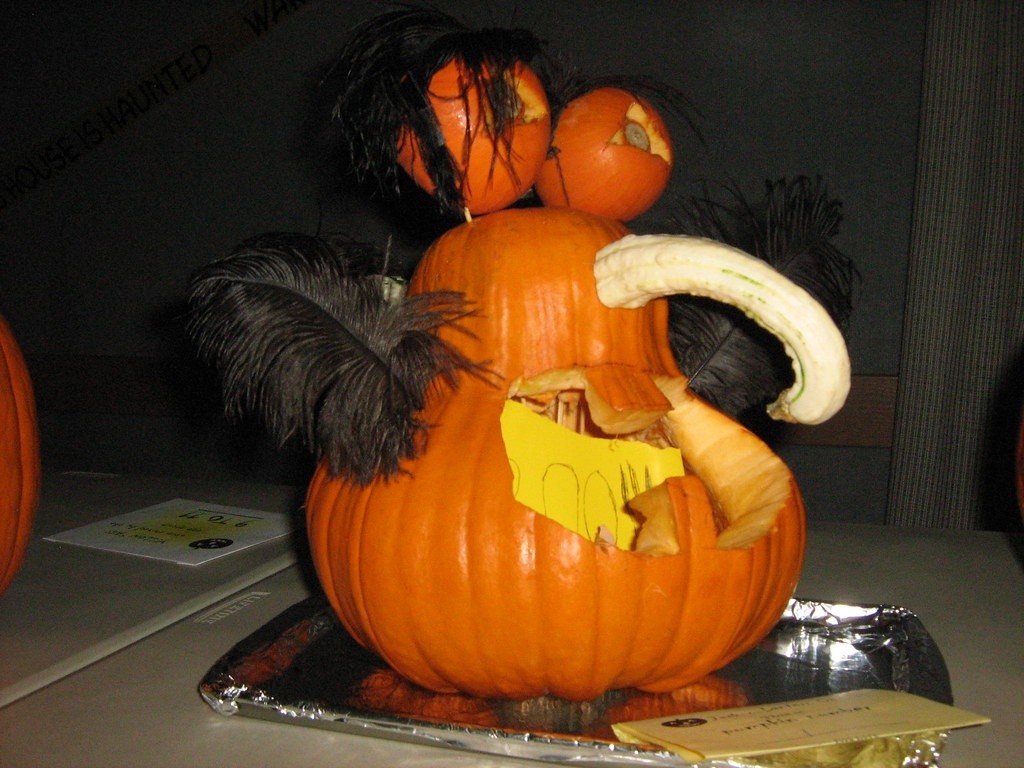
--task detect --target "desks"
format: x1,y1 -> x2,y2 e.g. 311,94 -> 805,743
0,523 -> 1023,768
0,470 -> 308,708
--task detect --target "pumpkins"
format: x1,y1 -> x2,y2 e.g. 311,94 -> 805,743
302,209 -> 805,697
0,315 -> 40,602
